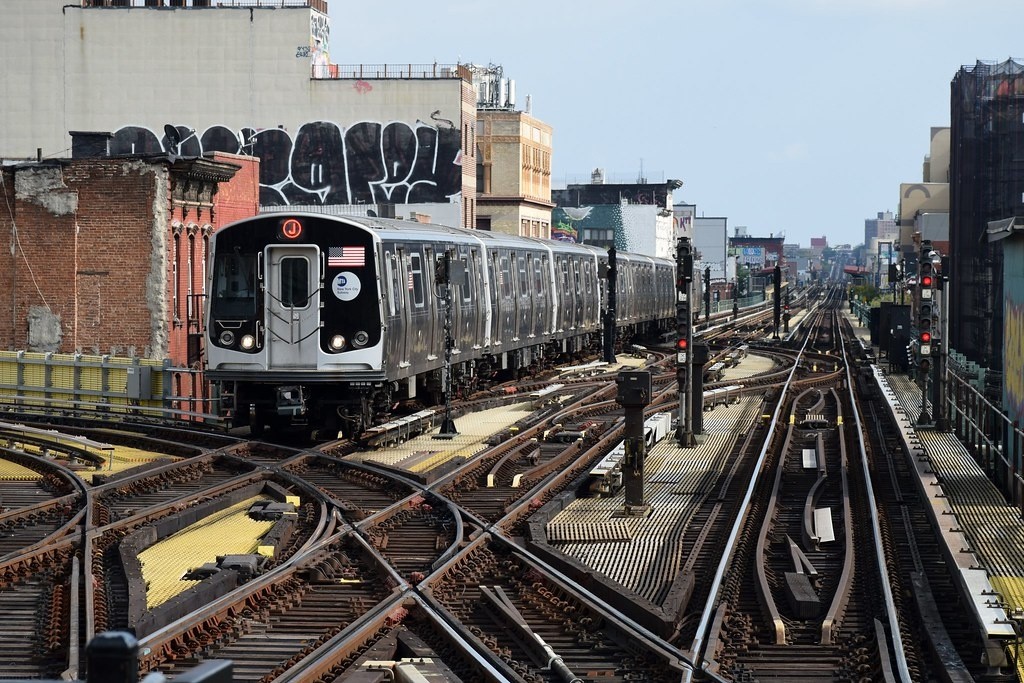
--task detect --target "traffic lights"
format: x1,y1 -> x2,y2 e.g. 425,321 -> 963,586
783,294 -> 789,313
674,240 -> 692,366
919,244 -> 933,358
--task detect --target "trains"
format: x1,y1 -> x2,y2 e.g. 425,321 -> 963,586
197,209 -> 703,443
